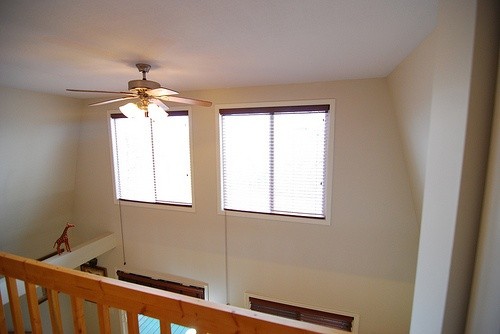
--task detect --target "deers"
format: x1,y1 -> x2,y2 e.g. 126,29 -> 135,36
53,222 -> 75,255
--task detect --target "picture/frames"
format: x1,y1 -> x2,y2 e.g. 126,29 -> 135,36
79,264 -> 108,304
35,249 -> 67,305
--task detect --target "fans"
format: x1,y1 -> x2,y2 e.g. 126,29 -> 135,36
65,63 -> 213,123
